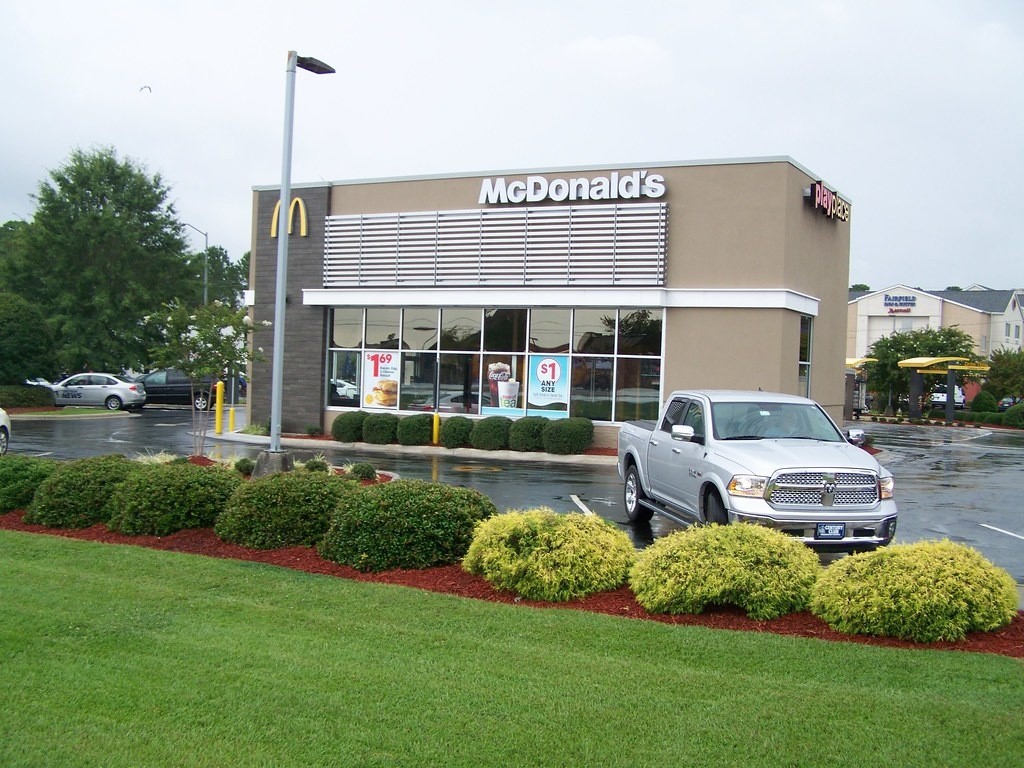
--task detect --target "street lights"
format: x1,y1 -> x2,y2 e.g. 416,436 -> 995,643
264,49 -> 335,476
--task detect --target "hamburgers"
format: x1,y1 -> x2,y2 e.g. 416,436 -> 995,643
373,380 -> 397,406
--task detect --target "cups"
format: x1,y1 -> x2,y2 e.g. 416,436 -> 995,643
487,363 -> 511,407
498,381 -> 520,408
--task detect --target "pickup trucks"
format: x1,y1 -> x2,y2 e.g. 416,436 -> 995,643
615,389 -> 897,556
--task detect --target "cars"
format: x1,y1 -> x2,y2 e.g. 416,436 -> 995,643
423,392 -> 494,410
45,372 -> 147,411
223,369 -> 247,395
929,382 -> 965,410
997,397 -> 1014,412
25,377 -> 52,387
133,368 -> 223,411
